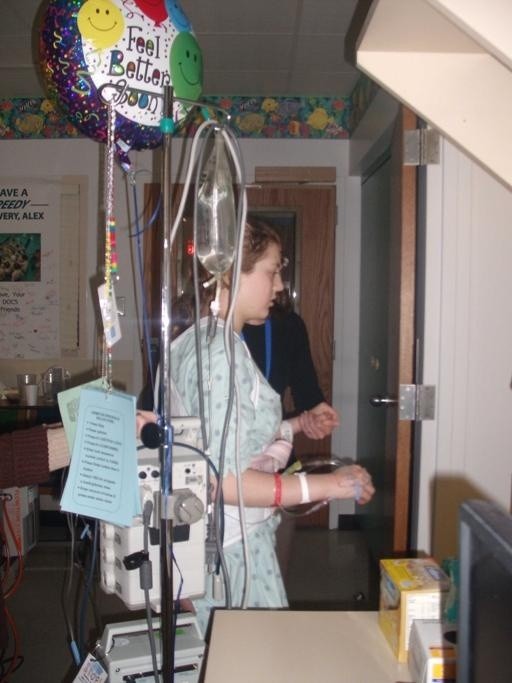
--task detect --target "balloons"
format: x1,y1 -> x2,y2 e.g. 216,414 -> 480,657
38,0 -> 205,151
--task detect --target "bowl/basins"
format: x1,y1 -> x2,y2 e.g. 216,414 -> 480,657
3,393 -> 18,403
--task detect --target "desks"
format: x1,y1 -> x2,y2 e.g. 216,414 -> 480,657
0,396 -> 59,429
197,606 -> 419,683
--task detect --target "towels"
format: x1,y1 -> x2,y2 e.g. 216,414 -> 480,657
0,422 -> 71,490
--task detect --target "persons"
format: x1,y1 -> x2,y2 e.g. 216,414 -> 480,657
0,236 -> 42,284
244,301 -> 340,590
151,213 -> 379,642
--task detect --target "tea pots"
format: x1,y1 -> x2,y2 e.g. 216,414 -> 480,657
42,366 -> 64,403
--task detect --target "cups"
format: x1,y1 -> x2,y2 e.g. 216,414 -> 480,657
16,373 -> 38,407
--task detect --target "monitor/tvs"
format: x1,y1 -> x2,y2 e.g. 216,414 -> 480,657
454,499 -> 512,683
